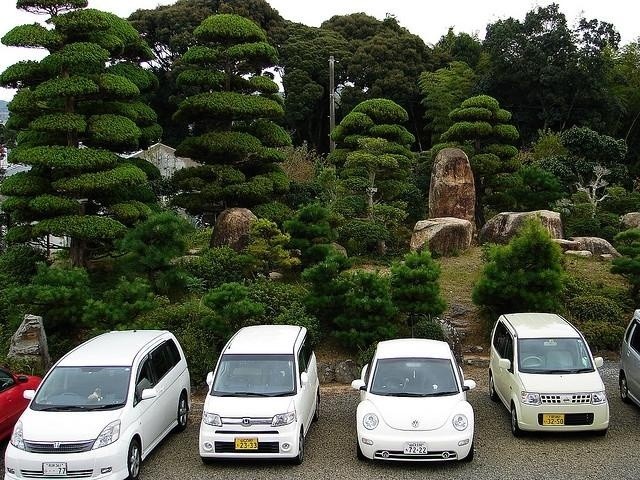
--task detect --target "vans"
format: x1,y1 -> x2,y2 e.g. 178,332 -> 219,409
3,330 -> 192,479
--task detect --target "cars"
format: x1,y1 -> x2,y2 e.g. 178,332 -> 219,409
351,338 -> 477,464
198,325 -> 321,465
489,312 -> 610,438
0,366 -> 43,442
617,309 -> 640,408
